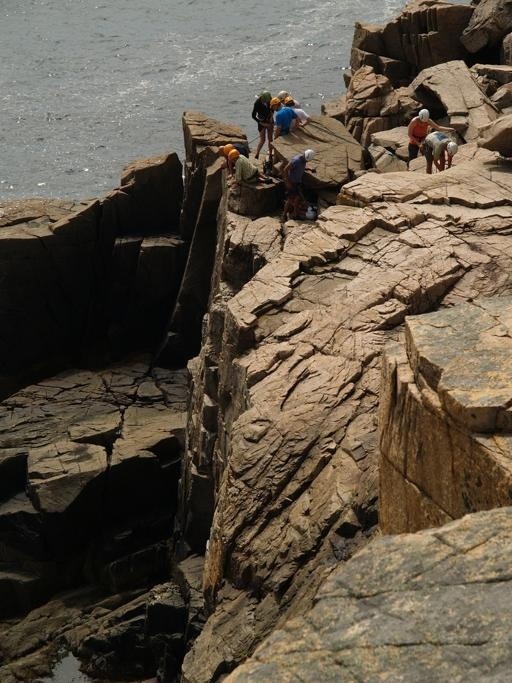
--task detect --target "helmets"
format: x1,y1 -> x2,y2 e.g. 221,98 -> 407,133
305,149 -> 314,161
447,142 -> 458,156
223,144 -> 239,161
419,109 -> 429,122
261,90 -> 293,108
306,206 -> 317,219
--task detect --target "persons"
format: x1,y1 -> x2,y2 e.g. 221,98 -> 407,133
251,89 -> 275,160
279,149 -> 316,223
283,96 -> 312,132
270,96 -> 302,139
227,148 -> 274,184
223,141 -> 249,181
278,90 -> 301,108
406,108 -> 457,171
423,129 -> 458,173
